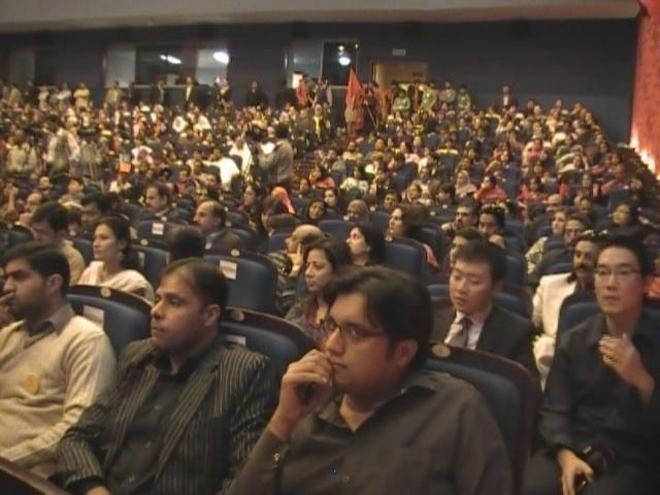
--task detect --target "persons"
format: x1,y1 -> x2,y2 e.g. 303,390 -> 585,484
0,72 -> 660,495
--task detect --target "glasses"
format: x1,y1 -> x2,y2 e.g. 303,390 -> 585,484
319,317 -> 387,342
594,268 -> 642,280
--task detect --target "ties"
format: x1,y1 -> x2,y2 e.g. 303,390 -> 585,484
447,318 -> 473,349
